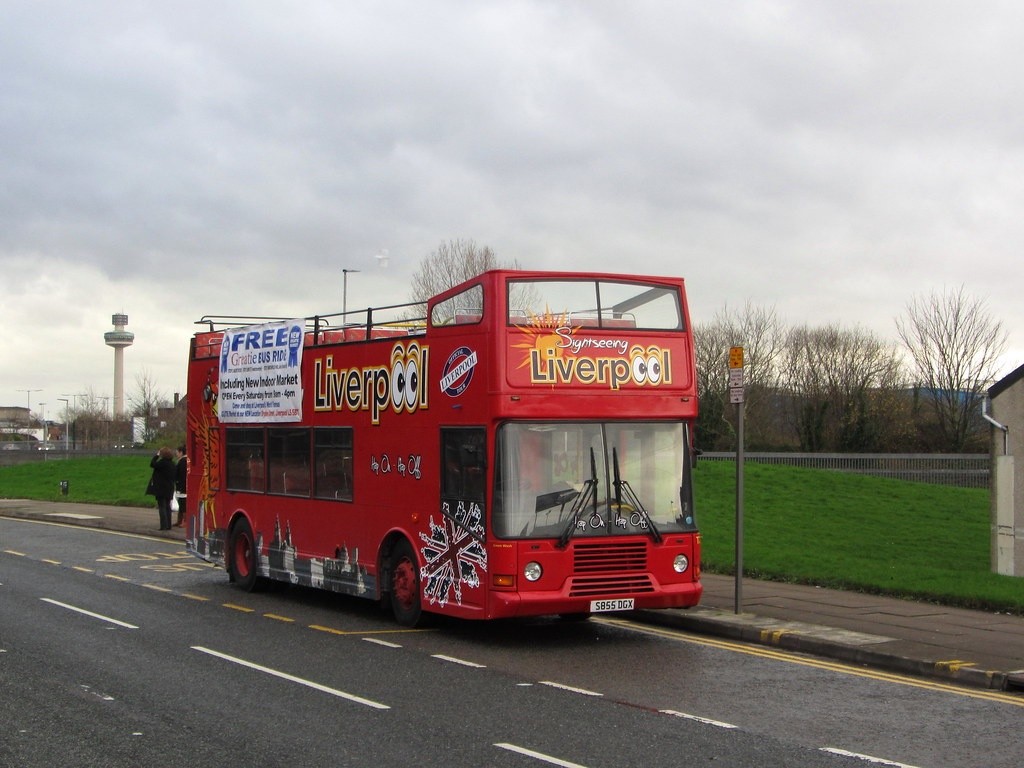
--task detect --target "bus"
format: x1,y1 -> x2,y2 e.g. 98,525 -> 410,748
183,269 -> 704,628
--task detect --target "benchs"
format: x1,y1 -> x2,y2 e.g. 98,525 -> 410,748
342,327 -> 408,342
196,332 -> 224,357
568,310 -> 636,328
453,307 -> 527,324
322,331 -> 344,344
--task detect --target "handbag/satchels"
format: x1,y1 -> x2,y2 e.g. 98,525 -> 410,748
170,492 -> 179,511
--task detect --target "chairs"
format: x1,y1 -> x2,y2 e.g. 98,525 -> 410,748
281,469 -> 309,495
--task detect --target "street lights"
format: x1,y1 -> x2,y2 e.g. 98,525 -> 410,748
17,389 -> 43,450
62,394 -> 88,449
343,268 -> 360,323
57,398 -> 69,460
97,396 -> 119,448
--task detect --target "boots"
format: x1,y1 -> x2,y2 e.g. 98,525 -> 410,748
173,512 -> 183,527
179,512 -> 186,528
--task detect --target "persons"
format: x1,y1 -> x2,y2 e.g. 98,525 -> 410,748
147,447 -> 175,529
173,445 -> 186,529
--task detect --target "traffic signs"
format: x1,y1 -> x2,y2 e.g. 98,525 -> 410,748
729,346 -> 743,403
